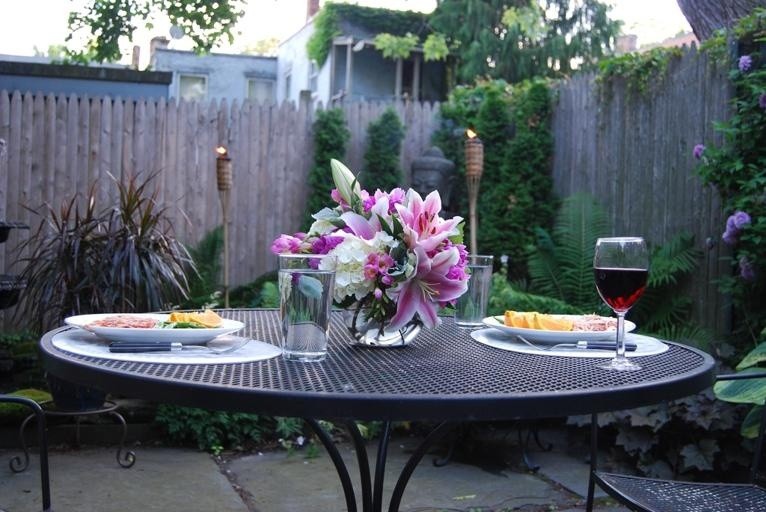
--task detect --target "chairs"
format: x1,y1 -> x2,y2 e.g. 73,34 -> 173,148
584,374 -> 766,512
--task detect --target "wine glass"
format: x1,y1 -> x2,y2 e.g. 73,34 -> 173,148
592,236 -> 651,373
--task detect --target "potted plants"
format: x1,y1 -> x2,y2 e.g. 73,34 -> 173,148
8,164 -> 208,411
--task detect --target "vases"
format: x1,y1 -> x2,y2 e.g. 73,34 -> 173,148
342,301 -> 423,347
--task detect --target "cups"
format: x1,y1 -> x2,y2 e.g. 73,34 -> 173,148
455,254 -> 494,328
276,252 -> 339,364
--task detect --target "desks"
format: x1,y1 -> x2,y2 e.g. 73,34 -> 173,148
39,308 -> 716,511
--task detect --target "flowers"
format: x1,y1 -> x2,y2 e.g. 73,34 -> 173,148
267,157 -> 474,343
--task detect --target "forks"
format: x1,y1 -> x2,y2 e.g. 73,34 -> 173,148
515,333 -> 638,352
106,337 -> 254,356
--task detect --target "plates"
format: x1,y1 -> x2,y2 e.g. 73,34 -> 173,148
483,311 -> 637,345
64,311 -> 245,346
